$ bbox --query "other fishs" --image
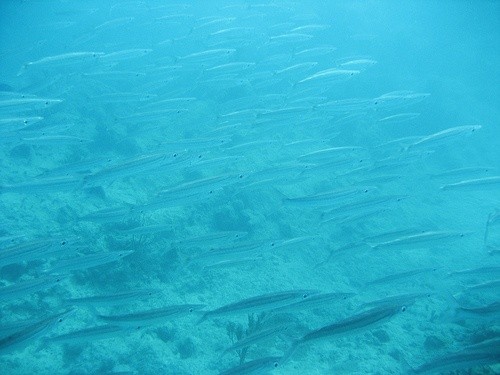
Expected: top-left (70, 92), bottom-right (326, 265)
top-left (1, 1), bottom-right (500, 375)
